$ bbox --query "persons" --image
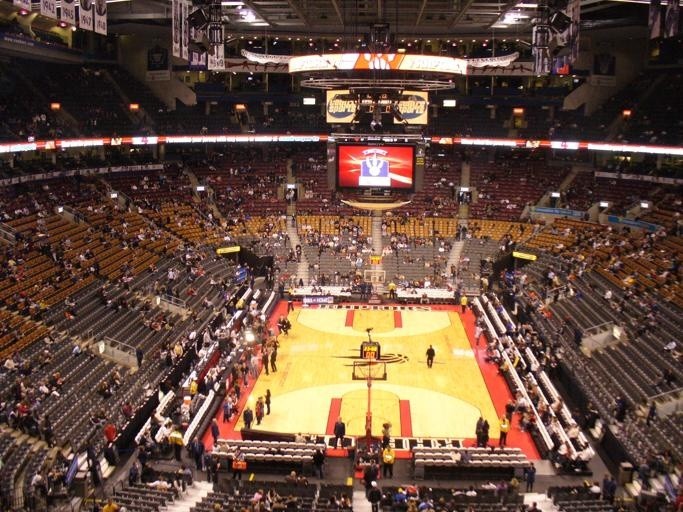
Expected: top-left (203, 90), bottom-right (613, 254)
top-left (1, 110), bottom-right (682, 511)
